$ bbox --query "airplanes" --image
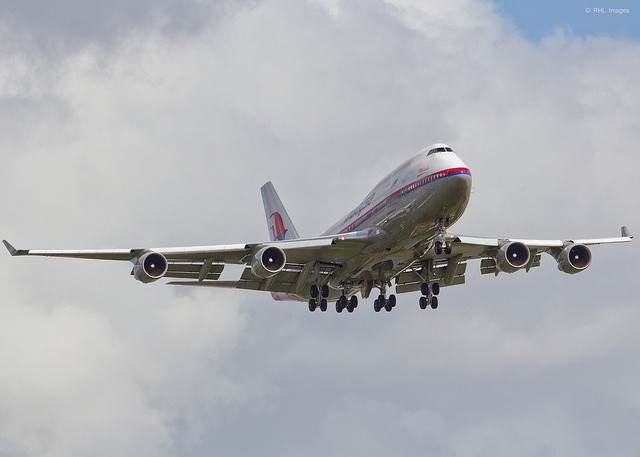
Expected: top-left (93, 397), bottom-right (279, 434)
top-left (1, 142), bottom-right (634, 311)
